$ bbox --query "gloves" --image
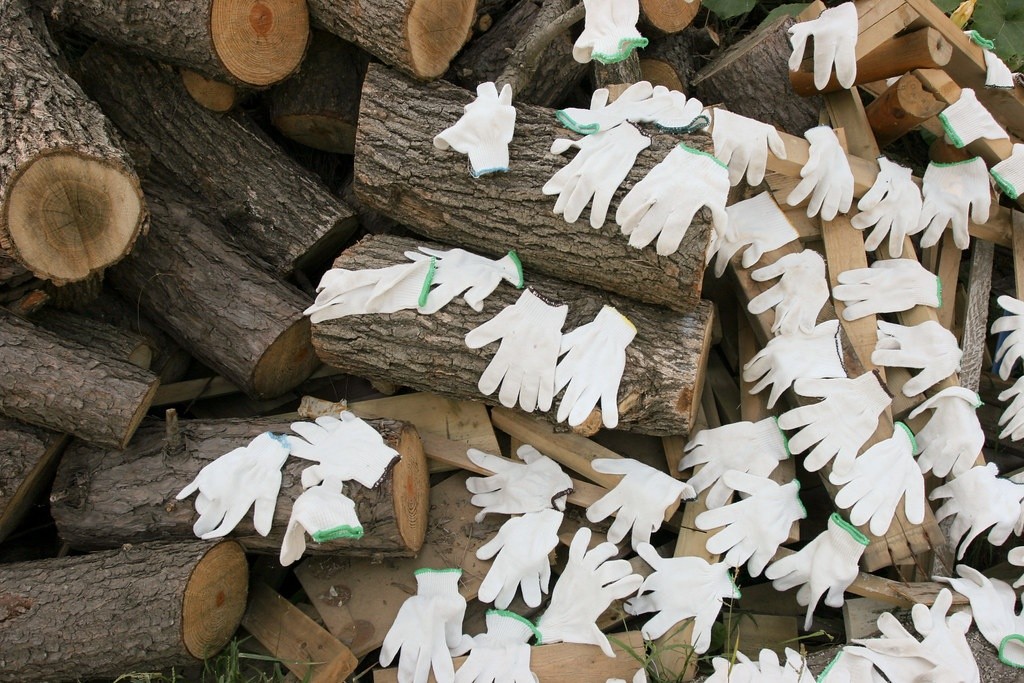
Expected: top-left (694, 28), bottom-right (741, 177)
top-left (301, 257), bottom-right (438, 325)
top-left (535, 29), bottom-right (1024, 683)
top-left (571, 0), bottom-right (650, 67)
top-left (535, 304), bottom-right (637, 429)
top-left (474, 509), bottom-right (565, 613)
top-left (403, 245), bottom-right (526, 315)
top-left (462, 443), bottom-right (574, 515)
top-left (378, 567), bottom-right (472, 682)
top-left (621, 542), bottom-right (743, 655)
top-left (690, 470), bottom-right (817, 579)
top-left (434, 81), bottom-right (518, 179)
top-left (533, 527), bottom-right (645, 659)
top-left (281, 410), bottom-right (403, 490)
top-left (278, 472), bottom-right (364, 567)
top-left (175, 431), bottom-right (291, 539)
top-left (453, 608), bottom-right (541, 683)
top-left (587, 458), bottom-right (699, 551)
top-left (783, 2), bottom-right (858, 91)
top-left (464, 285), bottom-right (568, 415)
top-left (763, 513), bottom-right (873, 633)
